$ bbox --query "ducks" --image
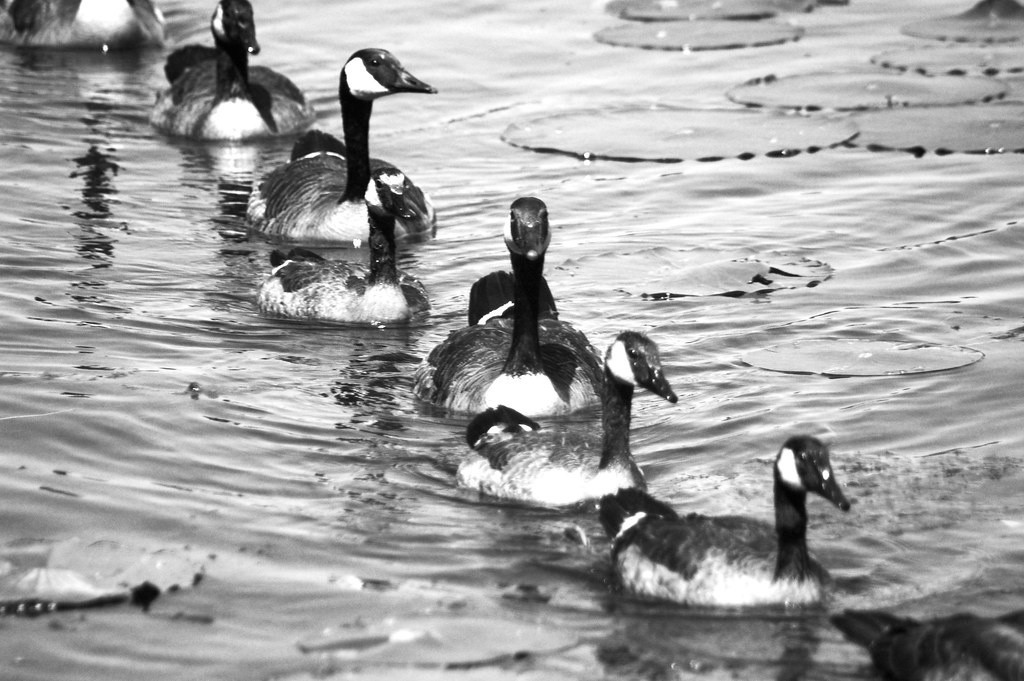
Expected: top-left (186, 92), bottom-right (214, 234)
top-left (828, 606), bottom-right (1024, 681)
top-left (0, 0), bottom-right (853, 611)
